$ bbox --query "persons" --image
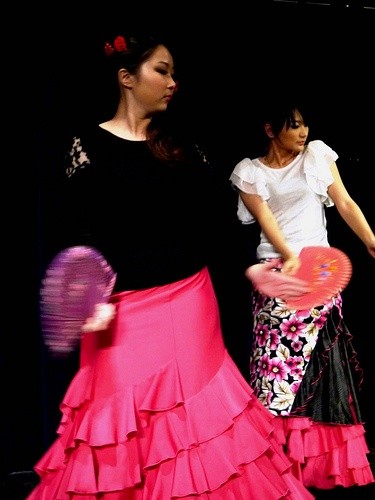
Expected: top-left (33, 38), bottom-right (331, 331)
top-left (226, 77), bottom-right (375, 490)
top-left (23, 21), bottom-right (319, 500)
